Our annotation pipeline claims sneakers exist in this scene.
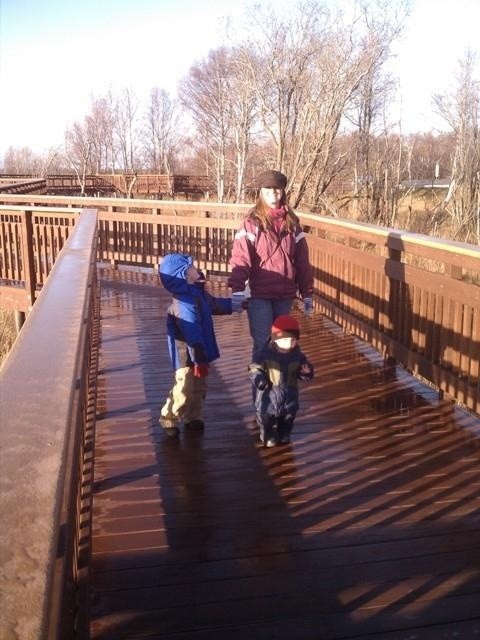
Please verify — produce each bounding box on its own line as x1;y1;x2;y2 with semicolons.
165;427;180;438
184;420;205;430
259;431;277;448
279;430;290;443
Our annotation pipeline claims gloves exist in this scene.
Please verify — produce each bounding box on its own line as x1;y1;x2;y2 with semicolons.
193;362;209;379
231;292;247;313
303;298;314;319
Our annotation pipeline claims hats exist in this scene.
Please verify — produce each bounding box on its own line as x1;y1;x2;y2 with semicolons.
256;170;287;190
270;315;300;341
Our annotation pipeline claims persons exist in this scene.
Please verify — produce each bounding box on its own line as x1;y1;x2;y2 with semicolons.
228;169;312;380
157;256;253;438
246;315;314;448
